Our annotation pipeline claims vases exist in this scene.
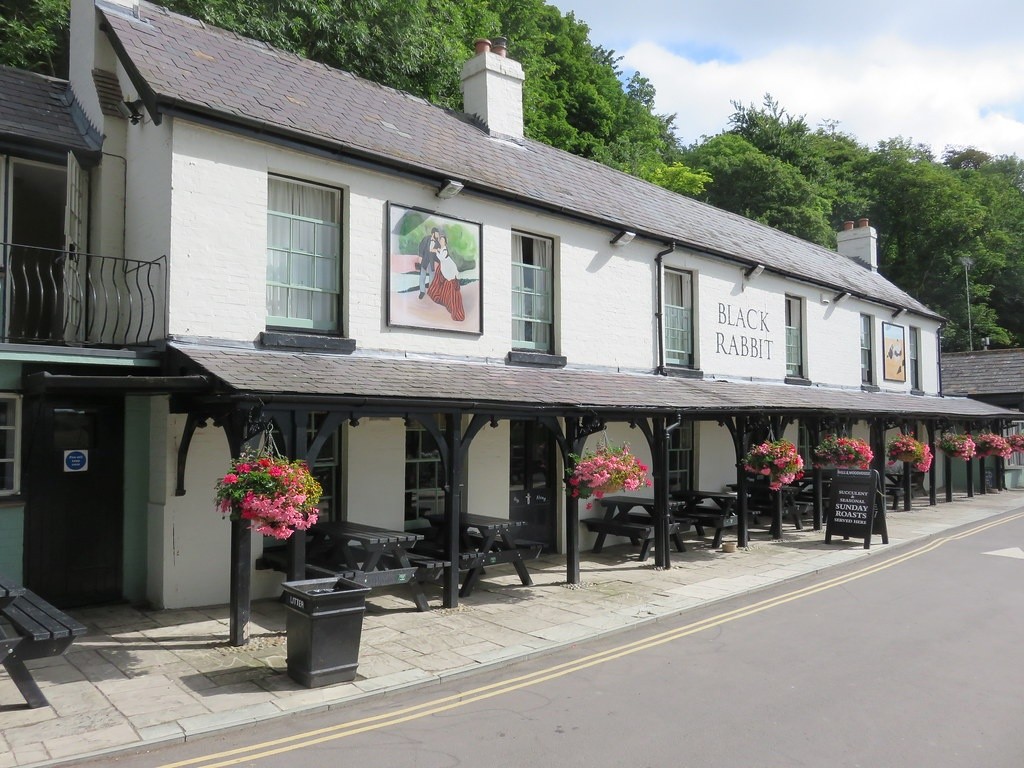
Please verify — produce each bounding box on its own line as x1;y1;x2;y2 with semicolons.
592;483;620;494
821;462;836;469
898;452;915;462
988;448;1002;455
946;450;961;457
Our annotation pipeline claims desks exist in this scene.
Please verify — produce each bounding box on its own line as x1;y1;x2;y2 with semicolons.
801;477;830;523
726;483;802;539
424;511;533;596
673;490;751;548
591;496;687;561
886;473;914;509
303;521;431;612
0;574;49;709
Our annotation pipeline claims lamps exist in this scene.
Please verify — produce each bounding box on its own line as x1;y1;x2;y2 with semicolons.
434;179;465;199
744;263;766;280
610;231;636;246
892;307;908;318
115;99;144;125
833;292;852;302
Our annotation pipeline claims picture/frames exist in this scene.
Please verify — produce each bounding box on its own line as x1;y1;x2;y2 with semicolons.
385;199;485;337
882;321;906;382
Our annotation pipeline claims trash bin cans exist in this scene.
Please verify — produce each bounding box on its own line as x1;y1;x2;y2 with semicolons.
281;576;372;688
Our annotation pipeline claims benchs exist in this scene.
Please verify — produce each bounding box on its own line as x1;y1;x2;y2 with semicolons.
885;486;904;492
798;496;830;504
673;510;724;528
255;546;363;581
0;588;87;662
911;483;917;488
793;501;813;512
696;505;763;515
408;539;483;570
748;503;789;516
465;530;545;561
629;512;698;524
581;517;655;539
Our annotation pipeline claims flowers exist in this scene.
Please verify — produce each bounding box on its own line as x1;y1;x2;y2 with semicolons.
934;429;976;463
812;432;874;470
563;438;652;509
212;442;324;542
1008;433;1024;454
976;432;1013;459
885;431;934;472
736;439;806;492
